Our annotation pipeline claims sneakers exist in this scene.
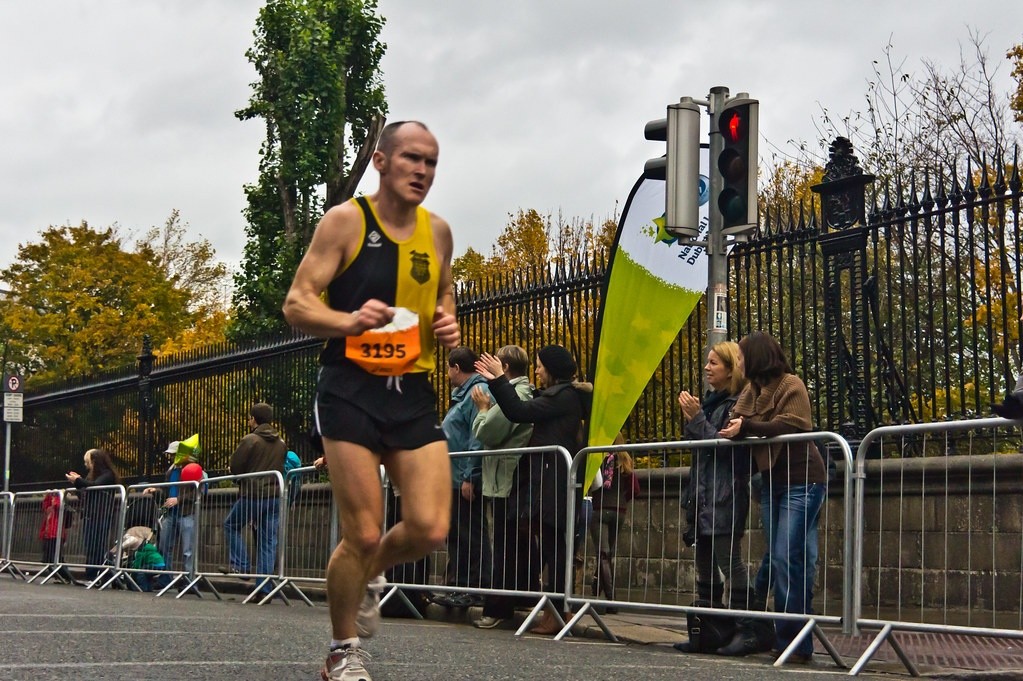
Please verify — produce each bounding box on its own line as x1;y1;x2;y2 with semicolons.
472;616;504;629
444;593;486;608
320;640;373;681
433;592;458;605
354;590;384;638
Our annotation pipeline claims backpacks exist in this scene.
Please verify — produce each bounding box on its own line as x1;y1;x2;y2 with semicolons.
283;450;302;504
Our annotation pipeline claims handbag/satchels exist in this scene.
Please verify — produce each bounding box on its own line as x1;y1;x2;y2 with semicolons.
684;599;738;654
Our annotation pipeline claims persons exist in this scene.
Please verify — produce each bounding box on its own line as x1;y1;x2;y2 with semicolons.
719;332;825;662
65;433;209;594
282;121;461;681
39;489;70;584
806;423;838;613
315;344;642;636
675;341;774;656
218;403;302;604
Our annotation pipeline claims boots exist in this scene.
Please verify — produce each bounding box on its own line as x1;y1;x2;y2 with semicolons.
596;551;618;614
672;581;725;652
590;567;604;596
715;586;760;657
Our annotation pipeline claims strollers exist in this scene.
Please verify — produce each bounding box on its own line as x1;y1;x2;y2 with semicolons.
90;491;174;592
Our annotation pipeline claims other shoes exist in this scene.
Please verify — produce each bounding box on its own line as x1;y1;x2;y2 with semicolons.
529;607;574;635
769;647;813;665
53;577;71;584
178;585;197;595
150;581;174;590
244;592;272;605
218;565;251;581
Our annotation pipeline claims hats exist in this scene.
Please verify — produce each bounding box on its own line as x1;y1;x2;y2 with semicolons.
537;345;577;379
164;440;180;454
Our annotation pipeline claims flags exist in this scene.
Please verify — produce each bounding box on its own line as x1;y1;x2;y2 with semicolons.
584;144;731;496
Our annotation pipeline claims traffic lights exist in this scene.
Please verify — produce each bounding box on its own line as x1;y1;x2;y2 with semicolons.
641;96;701;243
716;93;760;238
